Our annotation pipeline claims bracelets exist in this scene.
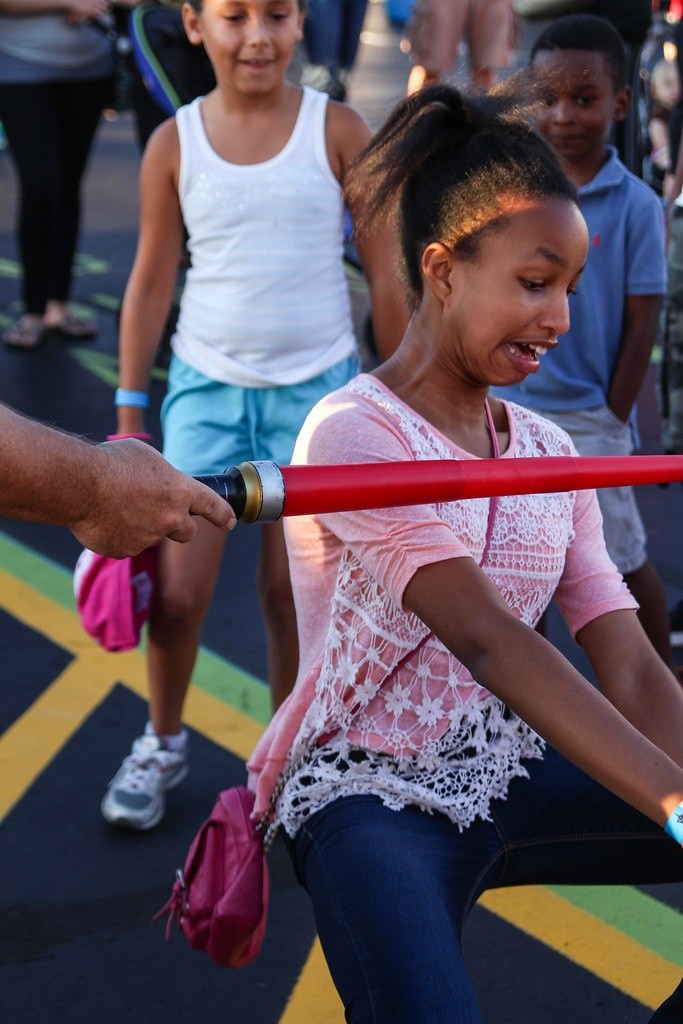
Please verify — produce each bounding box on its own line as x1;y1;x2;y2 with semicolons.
114;388;149;409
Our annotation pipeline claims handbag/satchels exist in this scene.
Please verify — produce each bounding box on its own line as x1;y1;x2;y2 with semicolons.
150;785;270;970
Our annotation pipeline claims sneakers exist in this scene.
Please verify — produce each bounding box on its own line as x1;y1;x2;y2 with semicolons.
668;599;683;647
100;722;199;831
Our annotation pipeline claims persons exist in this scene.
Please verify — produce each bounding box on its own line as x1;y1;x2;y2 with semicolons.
301;2;372;104
409;2;511;96
491;14;679;681
247;82;680;1022
1;404;237;559
93;2;418;827
1;0;119;351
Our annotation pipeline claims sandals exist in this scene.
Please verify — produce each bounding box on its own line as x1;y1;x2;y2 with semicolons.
3;315;44;350
45;317;93;341
